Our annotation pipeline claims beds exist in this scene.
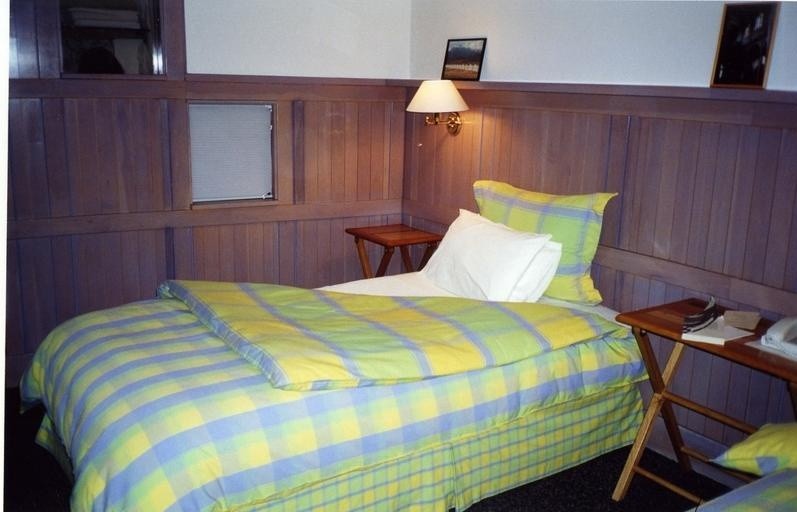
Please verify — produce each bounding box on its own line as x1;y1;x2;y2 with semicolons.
44;271;648;512
685;467;797;512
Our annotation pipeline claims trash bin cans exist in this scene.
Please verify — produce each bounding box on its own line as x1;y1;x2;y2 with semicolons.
761;315;797;360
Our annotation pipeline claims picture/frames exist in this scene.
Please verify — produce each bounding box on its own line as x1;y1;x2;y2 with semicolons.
708;0;781;89
440;37;487;82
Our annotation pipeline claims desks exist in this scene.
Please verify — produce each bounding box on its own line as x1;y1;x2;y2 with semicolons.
611;296;797;503
344;222;444;279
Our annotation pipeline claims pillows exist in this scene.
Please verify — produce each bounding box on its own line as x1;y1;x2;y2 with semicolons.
708;419;797;476
421;179;619;305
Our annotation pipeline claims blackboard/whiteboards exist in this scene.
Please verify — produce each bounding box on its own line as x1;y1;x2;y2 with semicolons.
186;102;275;204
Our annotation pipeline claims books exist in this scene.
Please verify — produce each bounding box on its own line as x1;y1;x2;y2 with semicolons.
680;294;726;348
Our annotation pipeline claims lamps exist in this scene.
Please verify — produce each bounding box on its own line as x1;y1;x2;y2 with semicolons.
406;80;470;136
